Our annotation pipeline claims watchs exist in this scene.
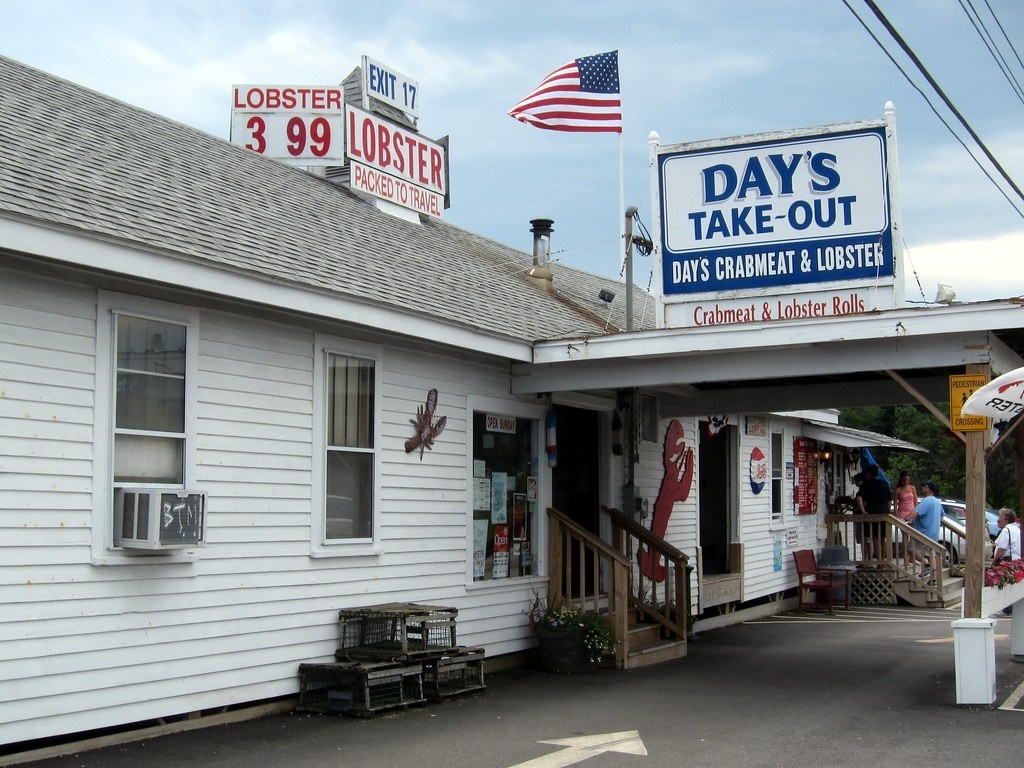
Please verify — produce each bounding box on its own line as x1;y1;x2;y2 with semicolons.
904;517;908;522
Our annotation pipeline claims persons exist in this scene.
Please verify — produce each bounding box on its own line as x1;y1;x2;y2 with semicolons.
893;470;918;567
852;465;892;570
991;508;1021;566
901;482;946;589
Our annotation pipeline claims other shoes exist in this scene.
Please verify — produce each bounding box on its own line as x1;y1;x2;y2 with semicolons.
927;580;937;586
919;566;936;577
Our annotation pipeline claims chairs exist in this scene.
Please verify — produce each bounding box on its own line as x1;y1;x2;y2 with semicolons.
793;550;852;614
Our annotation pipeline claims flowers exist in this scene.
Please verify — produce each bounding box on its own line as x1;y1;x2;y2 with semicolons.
984;557;1024;589
521;578;622;663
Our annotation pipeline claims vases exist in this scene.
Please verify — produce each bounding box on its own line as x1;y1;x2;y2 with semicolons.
535;630;599;674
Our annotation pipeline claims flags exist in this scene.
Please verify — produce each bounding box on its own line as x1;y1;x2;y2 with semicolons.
507;49;621;133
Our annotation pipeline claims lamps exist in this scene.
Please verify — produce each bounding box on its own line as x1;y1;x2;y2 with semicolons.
818;443;833;464
850;447;862;470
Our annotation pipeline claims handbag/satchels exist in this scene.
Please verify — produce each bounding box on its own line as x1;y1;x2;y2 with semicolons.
996;556;1012;566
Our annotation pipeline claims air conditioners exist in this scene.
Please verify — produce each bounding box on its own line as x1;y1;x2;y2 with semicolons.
115;487;207;548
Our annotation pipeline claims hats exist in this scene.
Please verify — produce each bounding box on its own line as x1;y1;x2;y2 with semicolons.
920;480;936;492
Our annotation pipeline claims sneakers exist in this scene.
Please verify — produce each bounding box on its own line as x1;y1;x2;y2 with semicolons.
995;611;1013;618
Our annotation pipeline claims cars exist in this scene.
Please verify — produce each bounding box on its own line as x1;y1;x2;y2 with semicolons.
888;498;1021;564
